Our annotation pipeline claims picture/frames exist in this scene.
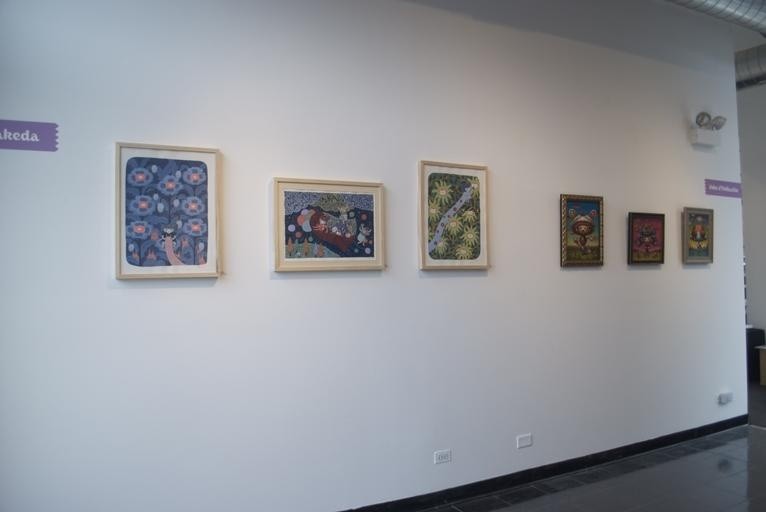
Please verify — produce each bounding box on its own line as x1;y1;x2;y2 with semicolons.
682;207;714;267
114;139;222;282
628;211;666;265
561;191;606;268
272;174;387;273
418;159;494;271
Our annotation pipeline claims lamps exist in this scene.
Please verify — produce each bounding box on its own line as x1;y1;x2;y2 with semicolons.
687;109;729;149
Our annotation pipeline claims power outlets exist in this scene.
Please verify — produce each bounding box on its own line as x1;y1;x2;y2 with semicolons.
431;446;453;466
